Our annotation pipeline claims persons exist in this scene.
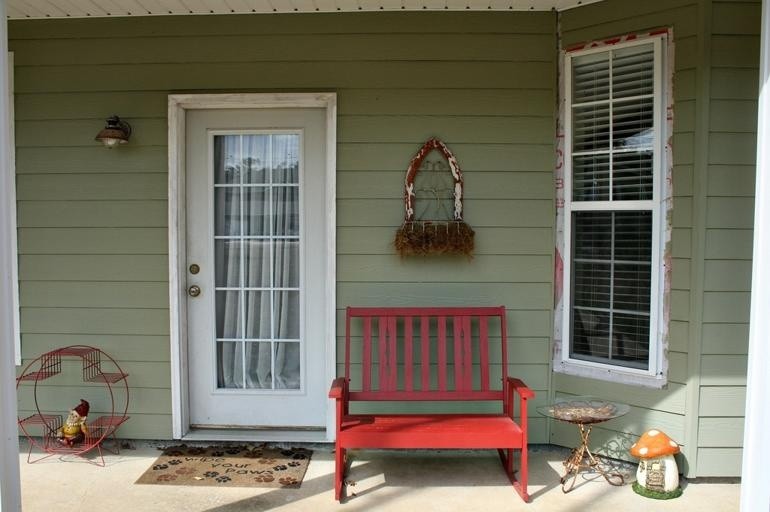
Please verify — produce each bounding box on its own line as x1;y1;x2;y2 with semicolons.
55;399;88;447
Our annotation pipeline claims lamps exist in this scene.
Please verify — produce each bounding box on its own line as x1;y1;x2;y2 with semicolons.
94;116;131;148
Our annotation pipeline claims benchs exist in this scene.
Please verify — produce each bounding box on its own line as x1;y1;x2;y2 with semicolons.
326;305;535;503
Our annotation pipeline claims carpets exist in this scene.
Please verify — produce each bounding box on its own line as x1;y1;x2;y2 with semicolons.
133;444;316;489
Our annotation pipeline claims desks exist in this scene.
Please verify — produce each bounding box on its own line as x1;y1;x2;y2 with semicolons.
537;398;631;494
